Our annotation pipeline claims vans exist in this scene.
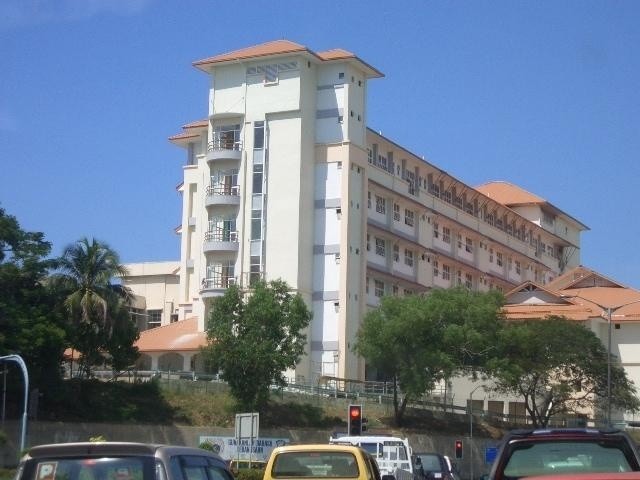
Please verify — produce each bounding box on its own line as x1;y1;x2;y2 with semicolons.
443;456;461;480
15;439;235;480
263;444;396;480
229;459;266;471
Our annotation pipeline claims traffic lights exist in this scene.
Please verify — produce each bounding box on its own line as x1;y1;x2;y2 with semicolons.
455;440;463;460
361;417;368;434
348;405;362;436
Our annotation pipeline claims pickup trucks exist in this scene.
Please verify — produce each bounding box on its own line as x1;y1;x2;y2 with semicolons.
480;426;640;480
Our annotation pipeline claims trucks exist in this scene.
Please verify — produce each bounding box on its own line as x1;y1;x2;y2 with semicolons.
299;433;414;480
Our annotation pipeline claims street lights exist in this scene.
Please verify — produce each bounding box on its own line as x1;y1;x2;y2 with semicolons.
558;293;640;429
469;383;489;480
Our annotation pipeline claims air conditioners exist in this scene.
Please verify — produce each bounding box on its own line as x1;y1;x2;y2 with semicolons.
231;186;239;196
230;232;238;242
228;278;236;288
233;141;242;151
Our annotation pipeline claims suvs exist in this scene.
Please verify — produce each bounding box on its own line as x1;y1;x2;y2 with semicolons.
413;451;456;480
